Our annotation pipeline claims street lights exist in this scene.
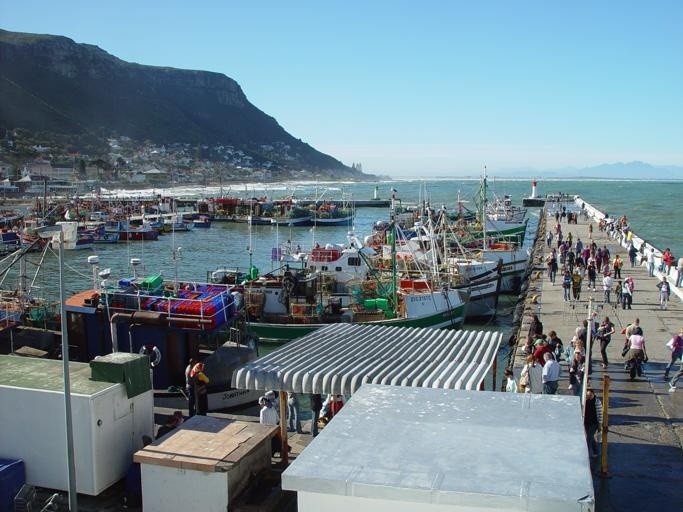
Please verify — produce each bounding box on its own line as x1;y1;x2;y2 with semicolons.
35;225;79;509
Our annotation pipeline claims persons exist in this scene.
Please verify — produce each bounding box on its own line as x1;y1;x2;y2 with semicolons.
157;411;184;439
501;191;682;459
184;357;197;419
190;362;210;417
257;390;346;459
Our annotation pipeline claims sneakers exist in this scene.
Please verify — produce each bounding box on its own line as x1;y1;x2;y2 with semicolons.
669;382;676;389
601;363;608;369
568;384;573;389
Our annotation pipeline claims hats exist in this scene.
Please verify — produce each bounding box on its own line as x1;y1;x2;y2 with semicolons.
534;339;542;346
571;337;579;342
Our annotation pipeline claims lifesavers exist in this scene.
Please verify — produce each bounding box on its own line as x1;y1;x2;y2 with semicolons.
138;344;161;368
